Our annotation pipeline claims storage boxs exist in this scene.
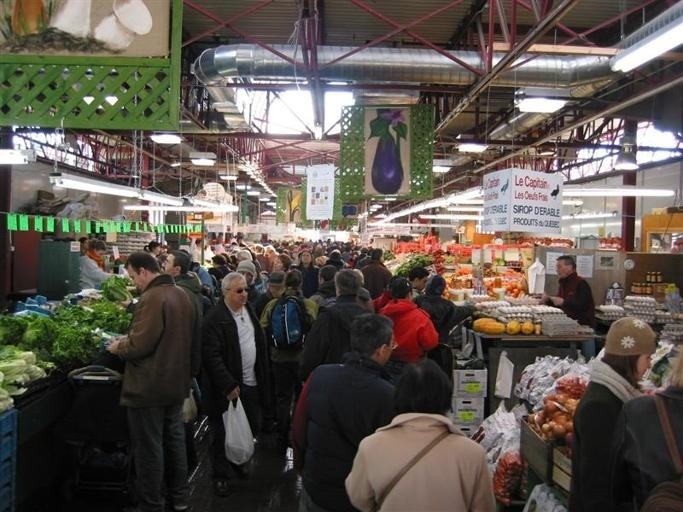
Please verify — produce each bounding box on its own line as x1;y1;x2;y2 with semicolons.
449;346;489;442
518;418;552;484
551;444;573;497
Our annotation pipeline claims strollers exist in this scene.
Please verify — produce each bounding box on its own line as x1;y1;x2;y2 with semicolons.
56;363;137;502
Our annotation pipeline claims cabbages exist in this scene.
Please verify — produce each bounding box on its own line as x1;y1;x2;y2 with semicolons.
100;275;133;304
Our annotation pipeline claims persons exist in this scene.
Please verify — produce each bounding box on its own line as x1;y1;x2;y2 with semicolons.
567;317;656;512
607;344;683;512
538;256;596;364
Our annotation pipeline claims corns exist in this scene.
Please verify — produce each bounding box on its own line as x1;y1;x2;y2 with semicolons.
506;321;521;335
472;318;495;331
479;322;504;334
522;322;533;335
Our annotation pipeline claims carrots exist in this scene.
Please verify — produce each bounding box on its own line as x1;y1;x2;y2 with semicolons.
557;377;585;399
492;453;524;506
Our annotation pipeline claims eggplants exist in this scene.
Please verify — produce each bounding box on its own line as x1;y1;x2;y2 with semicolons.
371;131;405;195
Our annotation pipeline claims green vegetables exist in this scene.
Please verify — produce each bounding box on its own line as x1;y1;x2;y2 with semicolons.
0;343;49;414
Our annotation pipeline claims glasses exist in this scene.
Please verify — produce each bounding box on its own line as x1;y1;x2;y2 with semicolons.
236;288;251;294
385;342;398;350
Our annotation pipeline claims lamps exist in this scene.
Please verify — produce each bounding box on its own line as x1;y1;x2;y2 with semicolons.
514;29;570;113
563;200;583;206
615;144;641;170
0;130;277;212
563;188;674;197
432;145;450;173
609;1;682;73
457;86;490;153
431;189;484;211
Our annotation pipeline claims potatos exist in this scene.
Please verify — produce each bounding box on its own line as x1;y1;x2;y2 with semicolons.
526;393;578;459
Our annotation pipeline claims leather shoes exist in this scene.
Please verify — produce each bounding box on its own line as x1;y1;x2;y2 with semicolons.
212;466;233;497
236;465;249;480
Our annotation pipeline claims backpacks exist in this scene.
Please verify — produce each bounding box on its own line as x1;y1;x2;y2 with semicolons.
267;295;312;353
639;393;683;512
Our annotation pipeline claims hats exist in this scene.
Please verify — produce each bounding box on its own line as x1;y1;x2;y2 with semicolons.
236;259;255;277
604;316;656;356
